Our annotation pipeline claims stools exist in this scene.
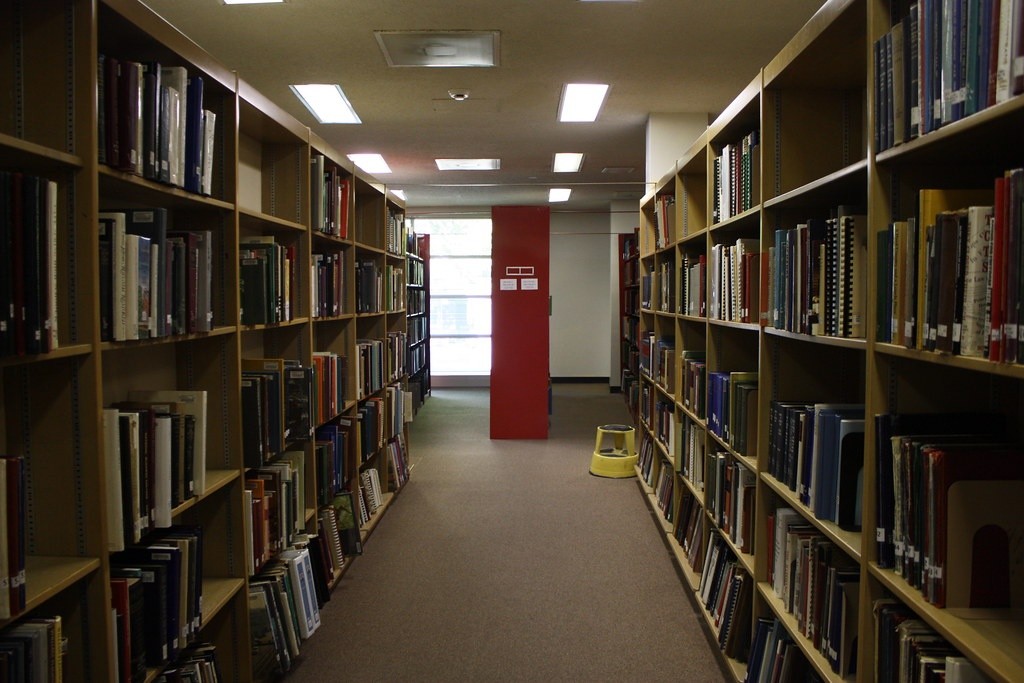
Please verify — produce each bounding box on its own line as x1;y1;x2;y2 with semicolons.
590;424;639;478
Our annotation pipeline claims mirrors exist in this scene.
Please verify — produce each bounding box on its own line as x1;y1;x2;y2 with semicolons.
0;0;1024;683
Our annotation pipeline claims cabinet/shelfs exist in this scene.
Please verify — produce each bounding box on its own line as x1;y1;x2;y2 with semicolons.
0;0;431;683
617;0;1024;683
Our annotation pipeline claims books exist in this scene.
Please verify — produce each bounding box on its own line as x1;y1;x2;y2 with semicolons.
0;54;430;683
621;0;1023;683
